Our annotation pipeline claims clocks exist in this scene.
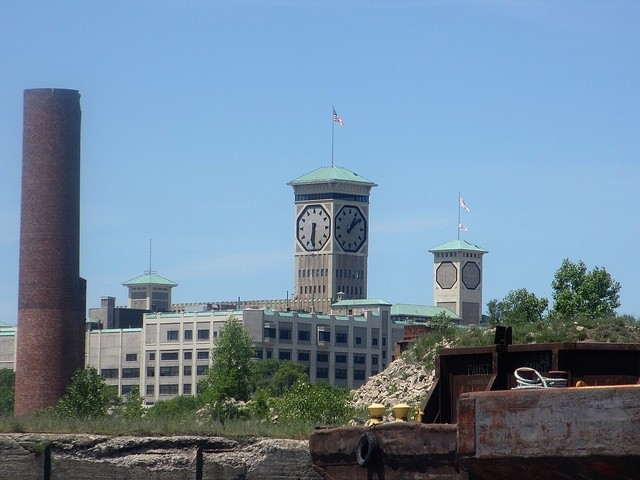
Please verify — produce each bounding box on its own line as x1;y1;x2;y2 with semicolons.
335;206;367;252
296;202;331;252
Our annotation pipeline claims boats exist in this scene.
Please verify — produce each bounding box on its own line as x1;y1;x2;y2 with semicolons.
309;326;640;480
